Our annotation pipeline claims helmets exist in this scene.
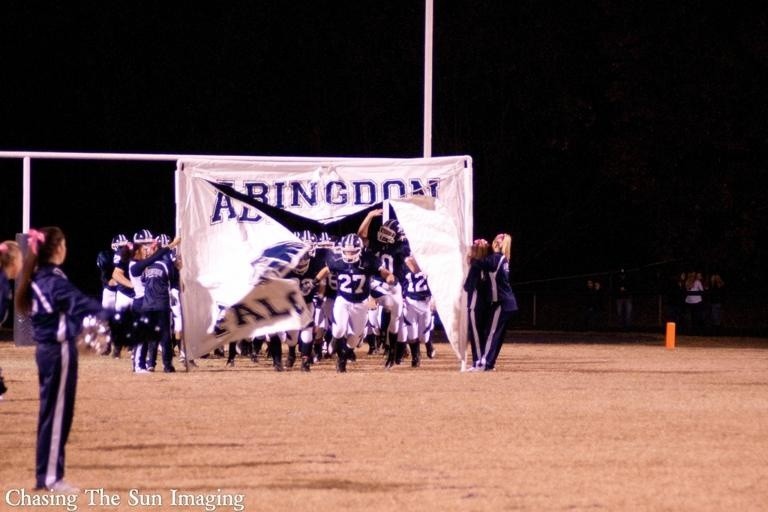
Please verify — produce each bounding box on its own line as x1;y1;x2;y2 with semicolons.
377;219;402;243
111;229;170;250
292;230;363;274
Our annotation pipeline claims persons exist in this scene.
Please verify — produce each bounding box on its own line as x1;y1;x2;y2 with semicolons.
16;227;111;493
0;239;23;398
582;268;728;329
92;209;522;375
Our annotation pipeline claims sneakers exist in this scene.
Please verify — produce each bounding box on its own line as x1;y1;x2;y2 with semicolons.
47;481;80;497
